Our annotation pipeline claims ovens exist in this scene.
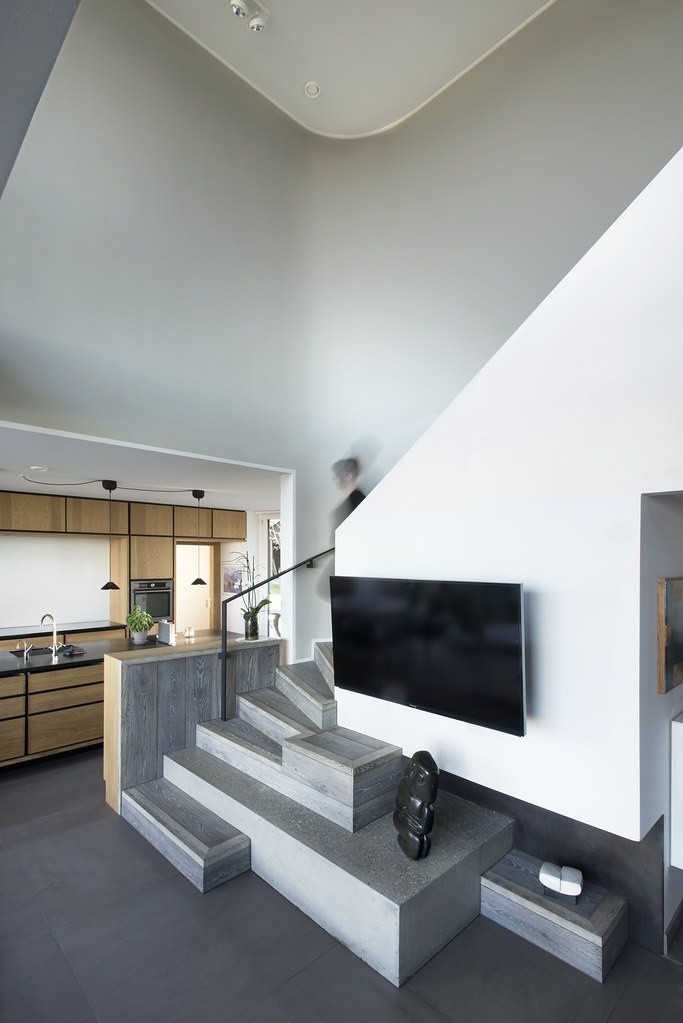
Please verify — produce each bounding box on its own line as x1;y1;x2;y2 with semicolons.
130;579;174;623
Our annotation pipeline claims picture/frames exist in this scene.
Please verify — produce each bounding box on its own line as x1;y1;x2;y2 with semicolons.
220;563;245;596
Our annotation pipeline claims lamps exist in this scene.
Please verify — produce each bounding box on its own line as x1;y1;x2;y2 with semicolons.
230;0;270;31
191;490;207;586
100;480;120;590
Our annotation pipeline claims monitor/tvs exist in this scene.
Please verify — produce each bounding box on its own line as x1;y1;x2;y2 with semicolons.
328;576;526;738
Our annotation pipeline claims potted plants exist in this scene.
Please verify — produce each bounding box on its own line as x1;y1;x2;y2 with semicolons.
217;551;272;640
126;606;155;644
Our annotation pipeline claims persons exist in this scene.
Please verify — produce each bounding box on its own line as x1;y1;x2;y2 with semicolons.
314;458;366;603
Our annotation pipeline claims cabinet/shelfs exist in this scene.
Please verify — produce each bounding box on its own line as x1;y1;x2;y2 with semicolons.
0;491;248;780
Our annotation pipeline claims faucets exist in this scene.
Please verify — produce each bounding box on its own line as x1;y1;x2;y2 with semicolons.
40;614;58;656
16;639;30;659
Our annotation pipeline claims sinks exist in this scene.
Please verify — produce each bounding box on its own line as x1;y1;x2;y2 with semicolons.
9;644;75;657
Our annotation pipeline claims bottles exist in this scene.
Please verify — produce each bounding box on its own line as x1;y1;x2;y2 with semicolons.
183;627;194;637
